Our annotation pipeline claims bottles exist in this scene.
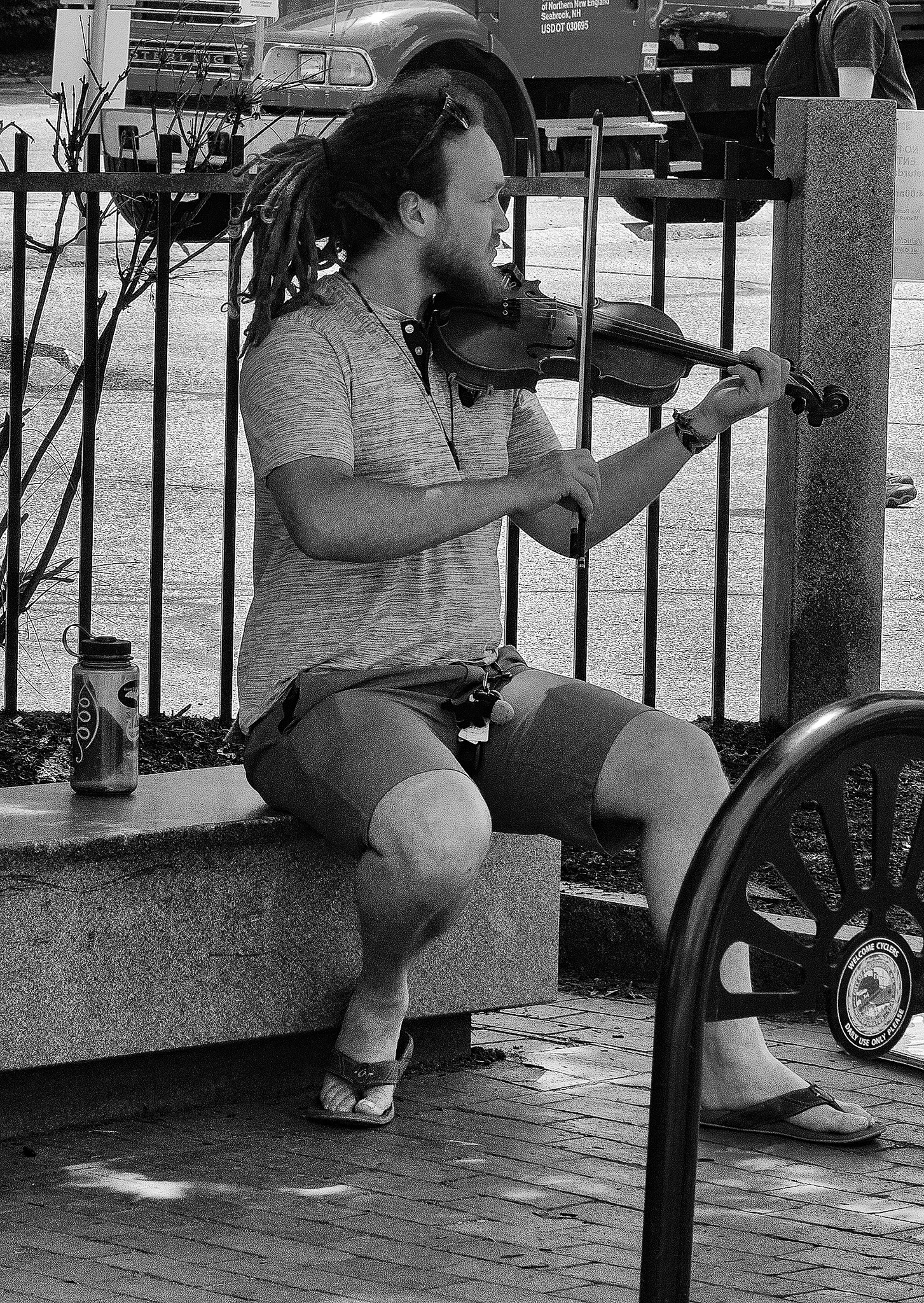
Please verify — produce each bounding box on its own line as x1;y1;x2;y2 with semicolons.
62;621;141;796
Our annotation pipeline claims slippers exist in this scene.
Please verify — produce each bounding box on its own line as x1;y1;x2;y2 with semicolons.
698;1077;887;1144
305;1029;414;1125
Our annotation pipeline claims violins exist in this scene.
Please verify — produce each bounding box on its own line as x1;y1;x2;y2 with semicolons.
430;261;850;425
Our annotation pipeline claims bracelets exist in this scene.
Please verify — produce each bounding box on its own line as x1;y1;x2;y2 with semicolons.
672;406;716;455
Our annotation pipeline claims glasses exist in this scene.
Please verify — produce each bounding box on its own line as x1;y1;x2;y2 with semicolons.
394;92;470;185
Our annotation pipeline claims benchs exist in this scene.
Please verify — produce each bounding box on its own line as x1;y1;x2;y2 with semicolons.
0;763;560;1146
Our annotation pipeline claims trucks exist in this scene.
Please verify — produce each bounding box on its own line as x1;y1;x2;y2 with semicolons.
103;0;924;244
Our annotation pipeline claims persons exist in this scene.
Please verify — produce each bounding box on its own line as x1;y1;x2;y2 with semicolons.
229;91;887;1146
